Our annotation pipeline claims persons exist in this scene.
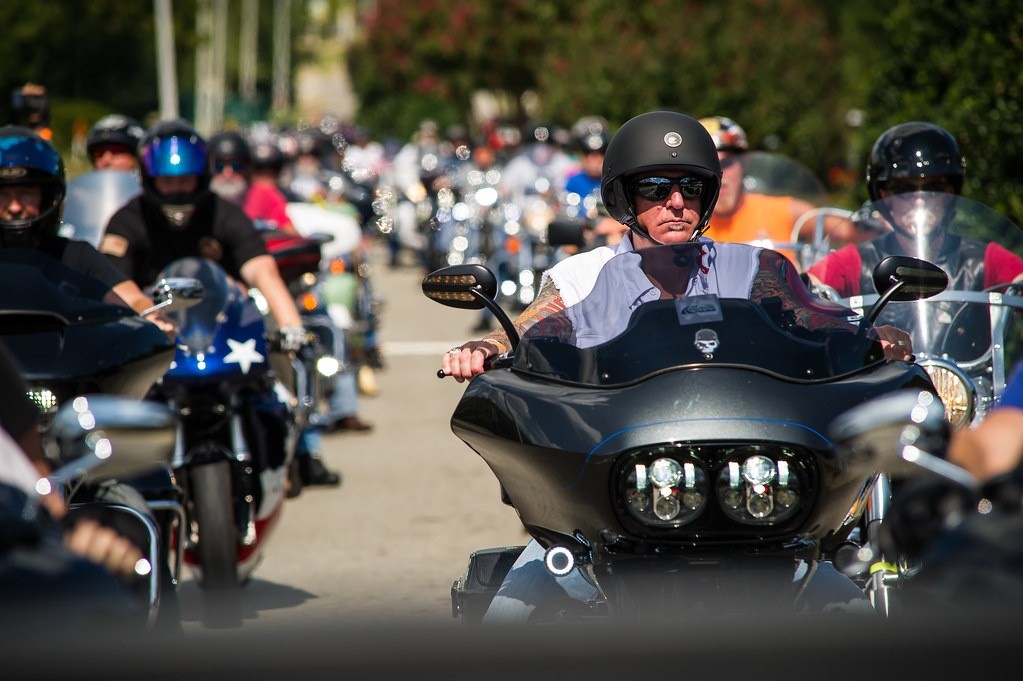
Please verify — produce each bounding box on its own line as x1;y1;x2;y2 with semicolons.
0;90;1023;637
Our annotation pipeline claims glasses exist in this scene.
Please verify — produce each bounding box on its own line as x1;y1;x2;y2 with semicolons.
883;180;952;199
214;163;248;175
635;175;708;201
721;156;735;169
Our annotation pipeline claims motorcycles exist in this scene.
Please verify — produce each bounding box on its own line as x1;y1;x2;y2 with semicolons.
793;190;1022;432
0;134;864;680
421;239;952;678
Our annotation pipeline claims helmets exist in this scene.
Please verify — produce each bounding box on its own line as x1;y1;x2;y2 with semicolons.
87;114;145;167
865;121;964;208
257;123;356;168
0;125;65;249
207;132;252;164
138;124;212;228
697;115;750;156
488;118;611;162
601;111;721;221
